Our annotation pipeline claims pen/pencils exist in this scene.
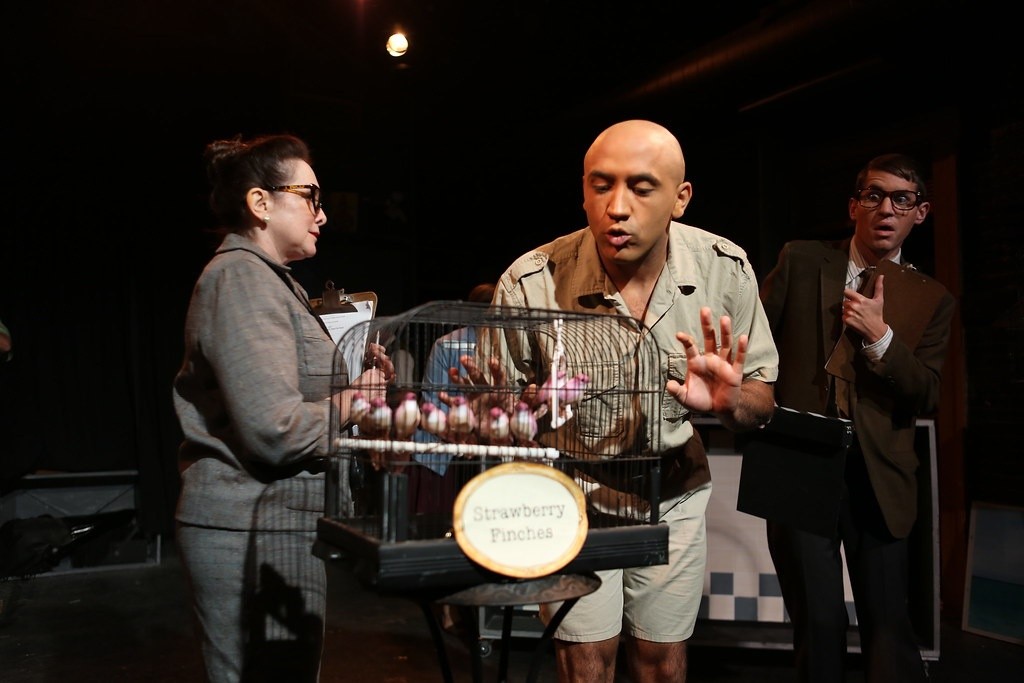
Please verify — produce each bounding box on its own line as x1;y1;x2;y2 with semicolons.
372;330;379;369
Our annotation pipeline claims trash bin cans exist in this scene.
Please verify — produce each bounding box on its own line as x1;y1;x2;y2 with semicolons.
0;487;165;683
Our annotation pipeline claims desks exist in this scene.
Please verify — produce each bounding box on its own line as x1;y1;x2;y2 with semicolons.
1;486;135;575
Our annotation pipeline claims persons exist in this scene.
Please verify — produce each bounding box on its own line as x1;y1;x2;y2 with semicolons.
173;133;397;683
413;120;779;683
737;153;956;683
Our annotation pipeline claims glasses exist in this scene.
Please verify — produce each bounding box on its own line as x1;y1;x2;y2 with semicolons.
261;184;322;208
856;188;922;210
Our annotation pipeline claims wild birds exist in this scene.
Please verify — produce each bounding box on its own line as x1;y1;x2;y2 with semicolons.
349;371;588;445
